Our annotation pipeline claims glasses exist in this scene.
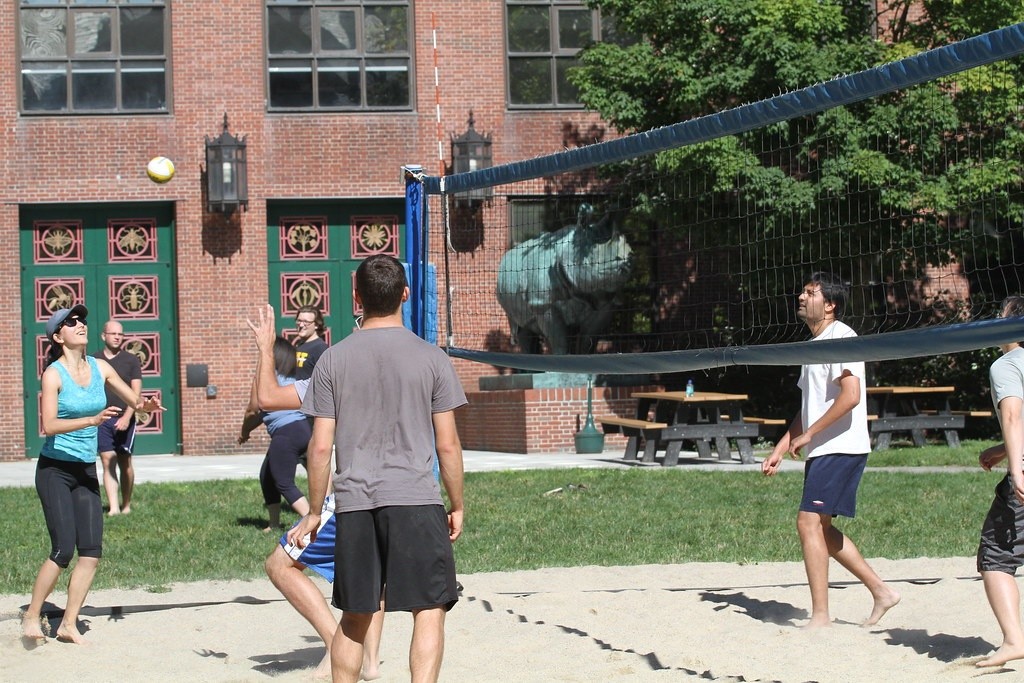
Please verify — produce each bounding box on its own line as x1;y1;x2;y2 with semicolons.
104;333;124;337
295;318;315;326
56;317;87;334
997;312;1004;320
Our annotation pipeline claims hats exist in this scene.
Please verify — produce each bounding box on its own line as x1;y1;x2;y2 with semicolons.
45;304;88;340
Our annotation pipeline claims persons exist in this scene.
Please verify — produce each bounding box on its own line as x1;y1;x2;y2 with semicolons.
246;304;385;680
975;295;1024;668
763;272;899;629
238;337;310;533
93;320;141;516
294;306;328;380
21;304;167;645
285;254;469;683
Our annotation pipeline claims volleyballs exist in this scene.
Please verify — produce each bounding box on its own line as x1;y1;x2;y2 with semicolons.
146;156;175;184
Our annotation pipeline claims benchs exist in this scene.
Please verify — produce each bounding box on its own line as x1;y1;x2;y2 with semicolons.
867;409;992;452
595;415;788;468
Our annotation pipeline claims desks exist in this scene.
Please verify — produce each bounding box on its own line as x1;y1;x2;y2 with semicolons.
866;385;965;452
624;391;760;468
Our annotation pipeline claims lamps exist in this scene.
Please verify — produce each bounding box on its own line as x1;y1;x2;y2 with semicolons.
450;110;495;209
204;111;249;224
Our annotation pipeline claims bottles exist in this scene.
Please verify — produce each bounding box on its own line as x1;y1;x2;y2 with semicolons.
686;380;693;397
